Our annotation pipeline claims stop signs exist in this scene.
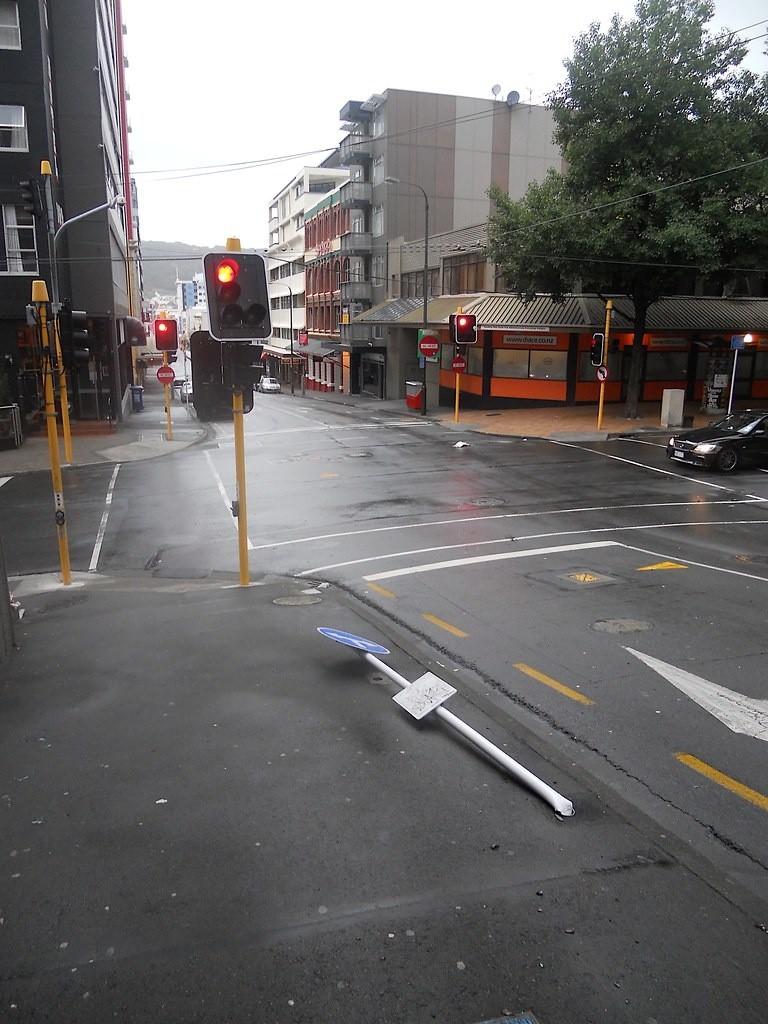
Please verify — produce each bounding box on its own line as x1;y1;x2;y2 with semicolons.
451;358;466;373
417;329;441;358
158;367;174;383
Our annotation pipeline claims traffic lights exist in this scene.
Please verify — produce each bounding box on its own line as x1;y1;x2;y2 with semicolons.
591;333;603;366
20;178;44;218
154;319;178;351
455;314;478;344
203;252;273;341
64;298;90;374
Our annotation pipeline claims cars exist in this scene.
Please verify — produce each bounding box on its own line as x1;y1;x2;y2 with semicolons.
173;371;188;386
666;411;768;474
179;382;193;405
252;383;257;391
259;378;282;394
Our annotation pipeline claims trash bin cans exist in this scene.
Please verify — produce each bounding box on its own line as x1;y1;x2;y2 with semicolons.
406;381;423;412
0;402;24;451
130;385;144;410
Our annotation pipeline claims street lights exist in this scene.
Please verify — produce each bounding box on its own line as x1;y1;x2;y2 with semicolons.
385;176;429;415
